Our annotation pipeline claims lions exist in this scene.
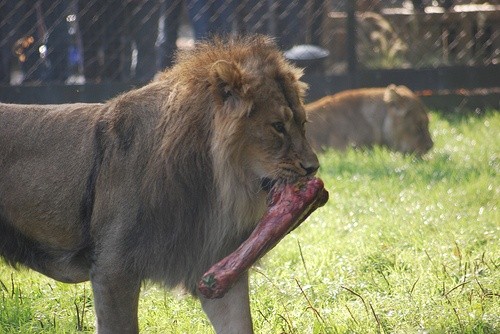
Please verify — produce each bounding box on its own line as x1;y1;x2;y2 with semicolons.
305;83;433;154
0;35;329;334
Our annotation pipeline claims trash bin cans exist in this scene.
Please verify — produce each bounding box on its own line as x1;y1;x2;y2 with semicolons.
284;44;329;99
